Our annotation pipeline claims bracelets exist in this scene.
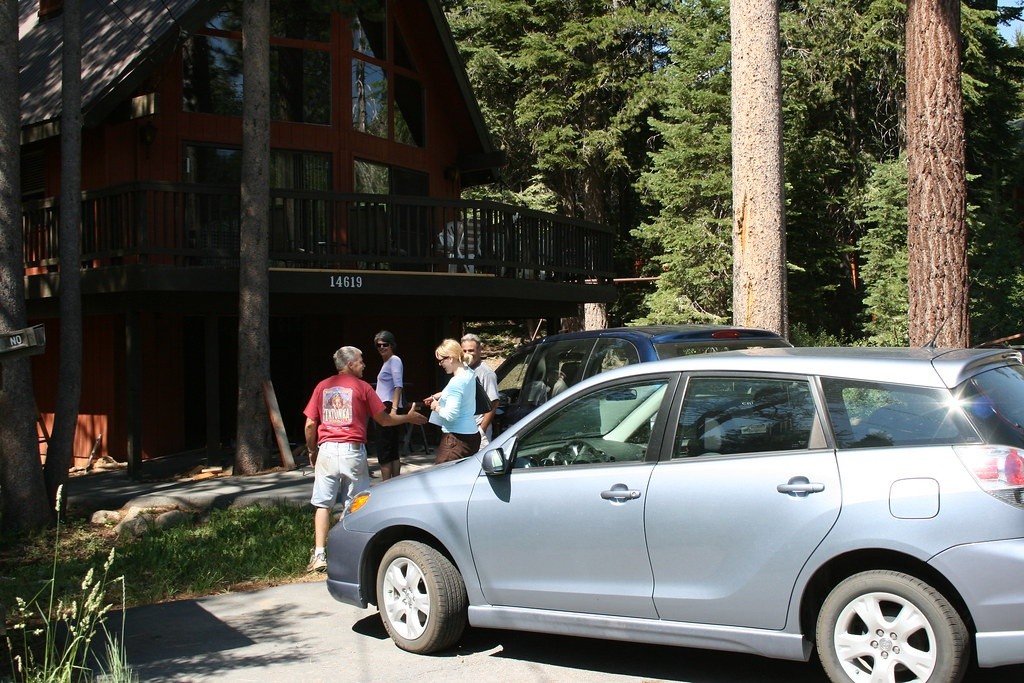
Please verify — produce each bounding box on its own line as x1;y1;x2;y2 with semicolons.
391;406;397;411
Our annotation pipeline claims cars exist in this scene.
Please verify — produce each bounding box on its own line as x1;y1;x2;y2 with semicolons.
323;343;1024;683
488;323;799;445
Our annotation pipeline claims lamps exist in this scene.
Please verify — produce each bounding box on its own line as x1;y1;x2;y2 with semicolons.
139;119;158;145
444;160;460;190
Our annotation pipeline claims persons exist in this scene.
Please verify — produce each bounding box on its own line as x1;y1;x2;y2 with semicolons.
303;346;428;572
423;333;501;451
552;363;577;399
375;331;404;480
428;339;482;464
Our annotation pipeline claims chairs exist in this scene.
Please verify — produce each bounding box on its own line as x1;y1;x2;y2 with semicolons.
430;217;481;274
751;387;787;423
551;362;581;398
691;409;739;457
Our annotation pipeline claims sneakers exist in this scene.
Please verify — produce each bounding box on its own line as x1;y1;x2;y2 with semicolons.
306;548;327;573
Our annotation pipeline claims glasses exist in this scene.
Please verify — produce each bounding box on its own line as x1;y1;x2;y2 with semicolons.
438;357;448;362
376;343;389;347
